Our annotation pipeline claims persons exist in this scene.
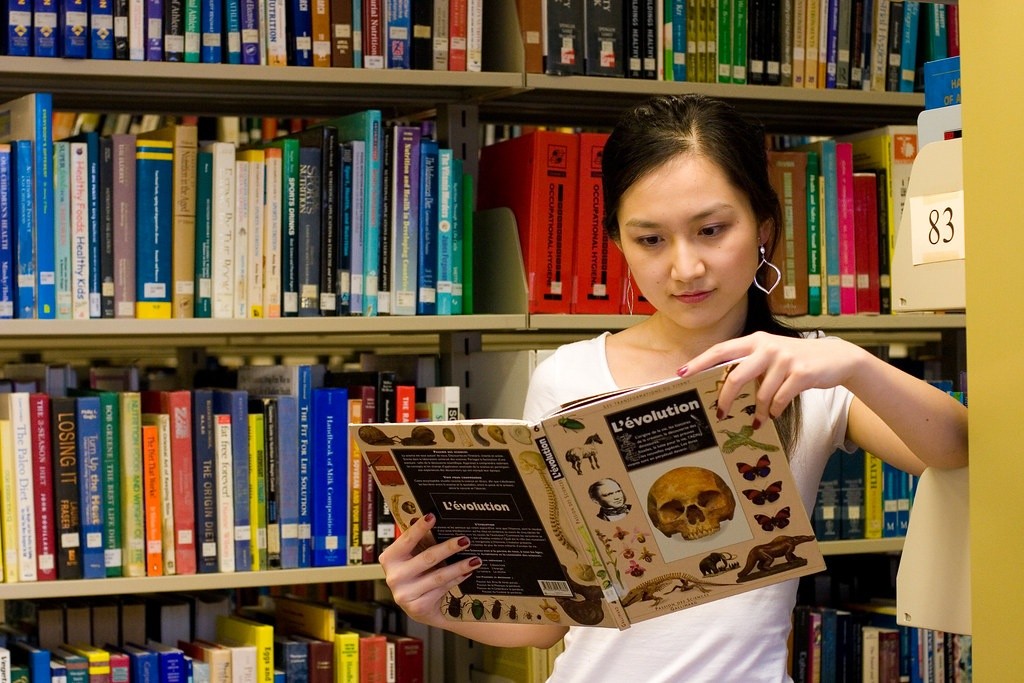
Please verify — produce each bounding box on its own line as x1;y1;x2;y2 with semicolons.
587;478;632;521
380;93;970;683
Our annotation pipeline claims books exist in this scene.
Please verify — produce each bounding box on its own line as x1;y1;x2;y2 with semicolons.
0;0;971;683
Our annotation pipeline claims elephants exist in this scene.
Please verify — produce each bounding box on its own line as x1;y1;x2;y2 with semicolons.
699;551;738;576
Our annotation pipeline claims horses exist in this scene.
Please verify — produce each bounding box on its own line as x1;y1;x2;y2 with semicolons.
564;432;604;476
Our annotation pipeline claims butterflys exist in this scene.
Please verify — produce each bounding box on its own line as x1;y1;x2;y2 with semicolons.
735;452;792;532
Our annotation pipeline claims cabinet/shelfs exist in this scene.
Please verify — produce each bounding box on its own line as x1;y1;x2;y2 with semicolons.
0;0;975;683
